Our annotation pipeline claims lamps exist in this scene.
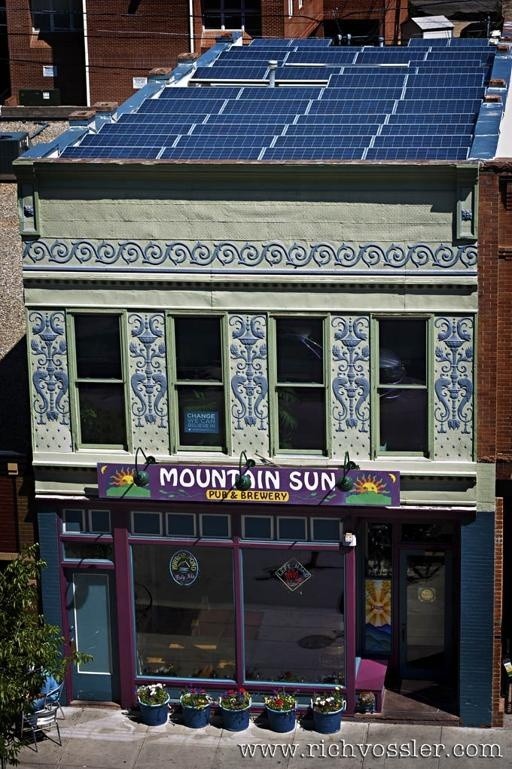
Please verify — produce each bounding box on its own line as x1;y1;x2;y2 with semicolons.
236;451;255;489
134;447;156;485
338;451;360;490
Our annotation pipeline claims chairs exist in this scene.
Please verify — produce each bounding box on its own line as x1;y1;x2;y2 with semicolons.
21;682;65;752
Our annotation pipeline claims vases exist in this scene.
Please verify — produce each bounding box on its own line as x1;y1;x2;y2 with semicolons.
130;695;346;734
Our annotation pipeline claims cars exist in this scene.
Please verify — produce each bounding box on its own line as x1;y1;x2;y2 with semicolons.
276;324;407;403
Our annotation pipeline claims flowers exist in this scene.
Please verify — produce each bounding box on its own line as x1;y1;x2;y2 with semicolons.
136;683;344;715
